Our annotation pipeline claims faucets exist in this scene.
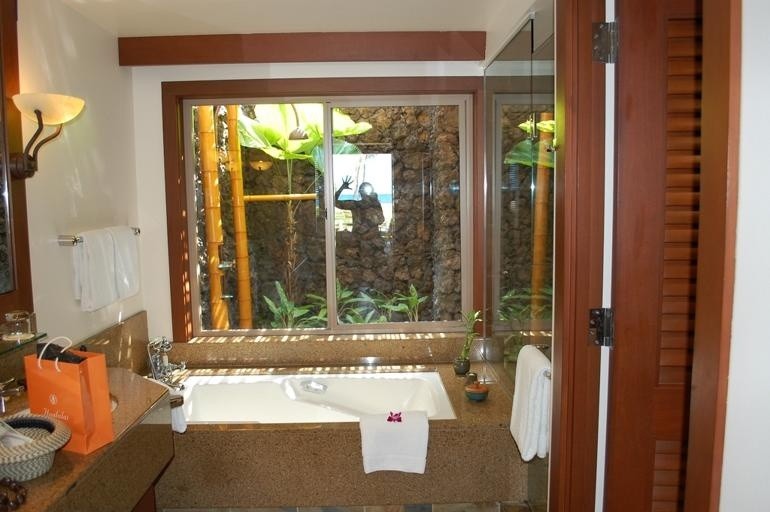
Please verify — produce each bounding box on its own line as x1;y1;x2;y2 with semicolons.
0;376;25;412
166;380;185;390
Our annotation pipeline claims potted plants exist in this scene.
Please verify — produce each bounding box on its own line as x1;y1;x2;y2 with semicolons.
452;309;483;373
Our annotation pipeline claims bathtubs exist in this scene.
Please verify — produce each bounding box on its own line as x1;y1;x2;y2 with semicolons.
171;372;457;425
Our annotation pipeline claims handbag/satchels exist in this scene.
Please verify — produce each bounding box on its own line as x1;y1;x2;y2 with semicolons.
20;335;116;457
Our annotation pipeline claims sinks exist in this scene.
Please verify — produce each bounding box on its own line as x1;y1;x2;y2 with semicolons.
12;392;119;414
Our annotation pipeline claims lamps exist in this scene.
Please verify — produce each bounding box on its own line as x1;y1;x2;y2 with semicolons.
9;92;86;181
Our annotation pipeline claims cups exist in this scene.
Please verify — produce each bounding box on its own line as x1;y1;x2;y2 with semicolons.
5;310;30;339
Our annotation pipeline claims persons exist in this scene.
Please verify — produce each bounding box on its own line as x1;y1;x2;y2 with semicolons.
335;175;385;238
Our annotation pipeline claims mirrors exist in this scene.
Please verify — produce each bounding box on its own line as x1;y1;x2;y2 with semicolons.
1;0;34;321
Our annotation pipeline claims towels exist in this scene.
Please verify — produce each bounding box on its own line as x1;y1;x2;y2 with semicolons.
359;411;429;475
146;375;188;434
510;343;554;464
72;229;117;312
103;226;143;300
